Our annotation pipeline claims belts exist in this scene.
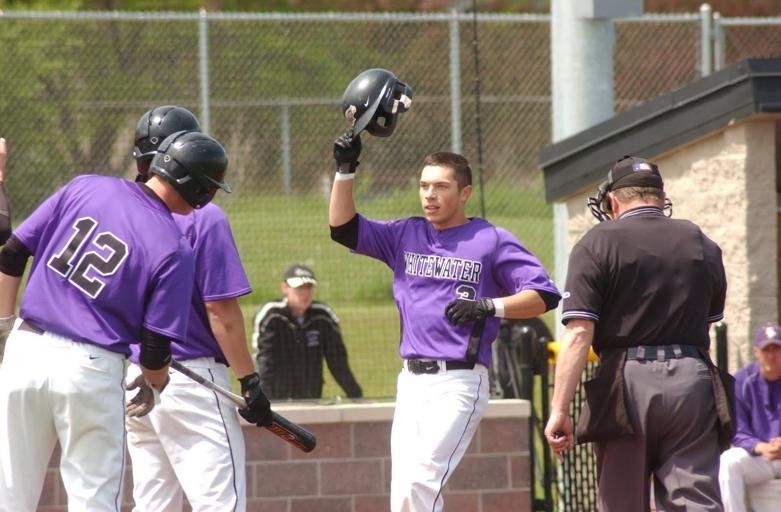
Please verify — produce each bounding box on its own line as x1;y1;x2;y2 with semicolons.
407;357;475;374
624;345;689;360
18;321;91;342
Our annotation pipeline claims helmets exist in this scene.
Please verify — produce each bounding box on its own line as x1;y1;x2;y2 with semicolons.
147;130;233;209
586;154;673;223
132;104;201;182
342;68;413;138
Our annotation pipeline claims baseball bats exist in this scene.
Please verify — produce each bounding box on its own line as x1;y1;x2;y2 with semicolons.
168;358;318;453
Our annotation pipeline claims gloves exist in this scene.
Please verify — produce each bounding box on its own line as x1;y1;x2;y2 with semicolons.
126;375;171;419
444;297;495;326
332;128;362;174
237;371;273;428
1;315;17;361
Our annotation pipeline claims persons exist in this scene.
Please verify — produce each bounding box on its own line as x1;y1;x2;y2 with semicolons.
0;137;13;246
249;263;363;404
715;320;781;511
328;129;563;512
544;153;728;511
122;105;270;512
0;130;233;512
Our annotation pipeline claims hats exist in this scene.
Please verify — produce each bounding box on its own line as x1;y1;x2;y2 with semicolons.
284;264;318;289
753;322;780;351
